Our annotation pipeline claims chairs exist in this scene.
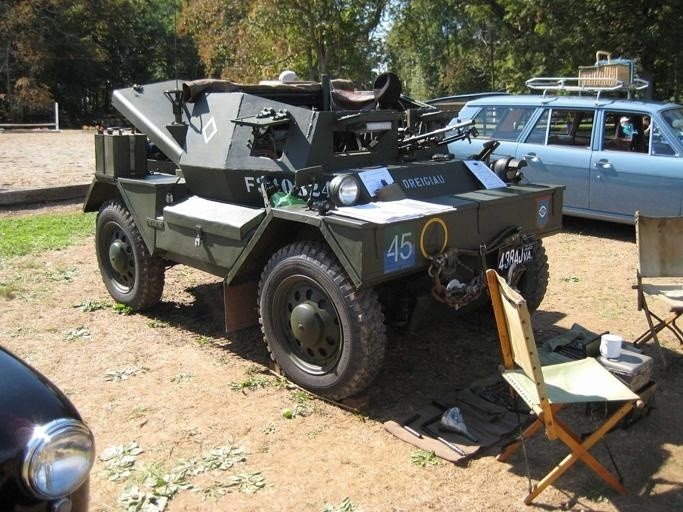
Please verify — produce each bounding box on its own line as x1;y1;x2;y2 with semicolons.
632;208;683;374
482;267;646;505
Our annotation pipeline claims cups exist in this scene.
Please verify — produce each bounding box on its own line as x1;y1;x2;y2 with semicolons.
599;334;622;359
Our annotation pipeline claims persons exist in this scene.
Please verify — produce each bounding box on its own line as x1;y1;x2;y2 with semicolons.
640;115;654;139
566;117;576;138
619;115;636;138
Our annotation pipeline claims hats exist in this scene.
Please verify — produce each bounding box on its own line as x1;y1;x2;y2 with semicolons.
619;116;629;122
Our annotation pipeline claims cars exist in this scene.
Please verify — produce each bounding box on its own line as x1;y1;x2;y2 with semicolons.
0;341;99;512
439;74;682;227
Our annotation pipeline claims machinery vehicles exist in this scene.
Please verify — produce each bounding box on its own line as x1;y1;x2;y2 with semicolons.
77;67;572;402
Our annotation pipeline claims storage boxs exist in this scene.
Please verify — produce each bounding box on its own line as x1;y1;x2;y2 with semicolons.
585;348;659;427
